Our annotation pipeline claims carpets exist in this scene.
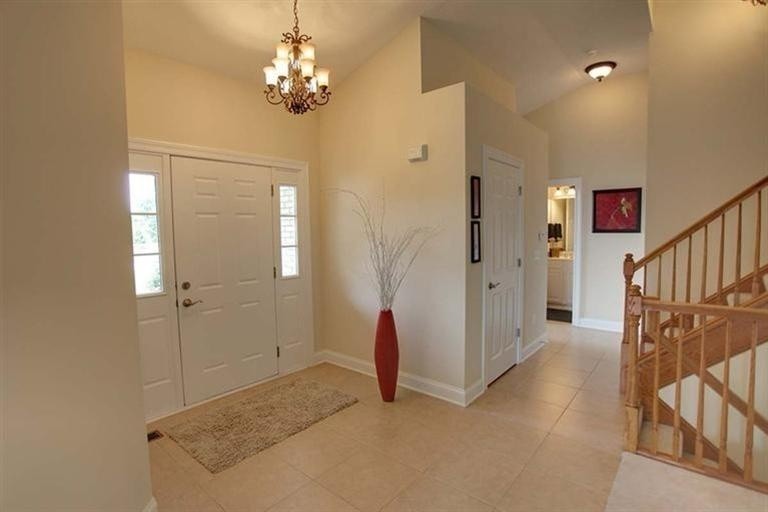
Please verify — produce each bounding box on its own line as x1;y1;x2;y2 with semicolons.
165;377;359;475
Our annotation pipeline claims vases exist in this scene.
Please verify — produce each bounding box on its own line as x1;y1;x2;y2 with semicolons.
373;309;401;402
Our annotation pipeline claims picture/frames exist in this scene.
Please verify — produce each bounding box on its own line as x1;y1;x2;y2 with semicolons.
471;221;481;263
591;186;643;234
470;174;482;219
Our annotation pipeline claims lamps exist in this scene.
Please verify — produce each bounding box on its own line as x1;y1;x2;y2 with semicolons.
567;186;576;195
555;187;563;196
262;0;332;115
584;60;617;82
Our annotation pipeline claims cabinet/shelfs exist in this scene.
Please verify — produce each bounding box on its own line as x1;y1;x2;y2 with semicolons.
547;260;573;311
